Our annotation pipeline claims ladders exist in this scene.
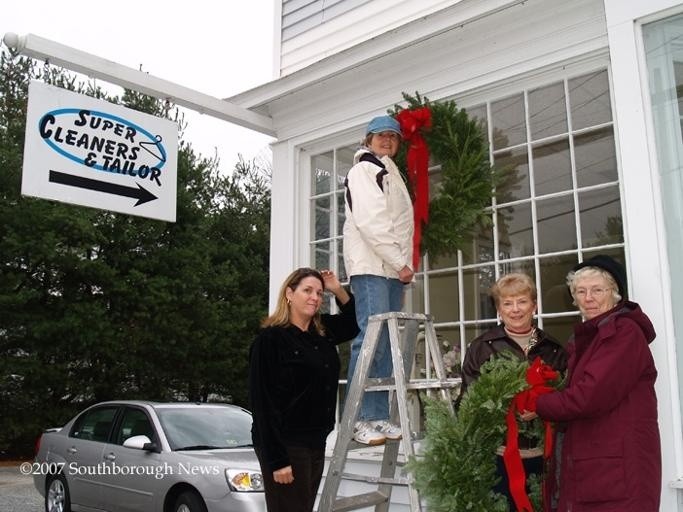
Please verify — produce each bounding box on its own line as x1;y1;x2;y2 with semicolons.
317;311;462;512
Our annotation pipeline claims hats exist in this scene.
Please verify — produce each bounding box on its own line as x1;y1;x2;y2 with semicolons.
365;116;403;137
573;254;627;303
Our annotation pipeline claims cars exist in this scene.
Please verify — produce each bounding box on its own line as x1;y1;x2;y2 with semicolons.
31;400;266;511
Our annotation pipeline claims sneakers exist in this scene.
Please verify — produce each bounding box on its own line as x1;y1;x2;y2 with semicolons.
369;418;405;441
352;420;388;446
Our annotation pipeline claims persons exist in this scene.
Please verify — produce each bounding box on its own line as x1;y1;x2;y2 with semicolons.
343;116;406;446
249;268;357;511
520;251;661;512
450;271;567;511
